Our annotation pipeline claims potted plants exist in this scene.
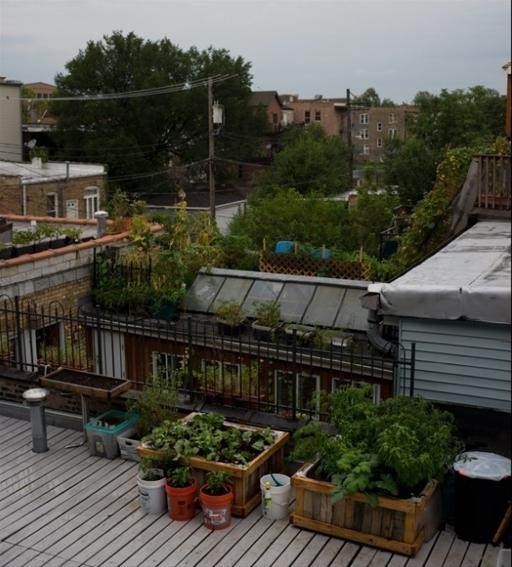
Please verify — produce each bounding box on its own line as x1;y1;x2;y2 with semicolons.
116;249;193;323
0;220;86;261
208;292;359;353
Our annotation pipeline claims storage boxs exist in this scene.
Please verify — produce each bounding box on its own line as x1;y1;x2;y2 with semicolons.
83;409;139;461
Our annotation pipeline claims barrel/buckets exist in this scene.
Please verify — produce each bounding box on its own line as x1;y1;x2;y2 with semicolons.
260;473;295;520
199;483;234;530
165;478;199;521
136;473;165;514
